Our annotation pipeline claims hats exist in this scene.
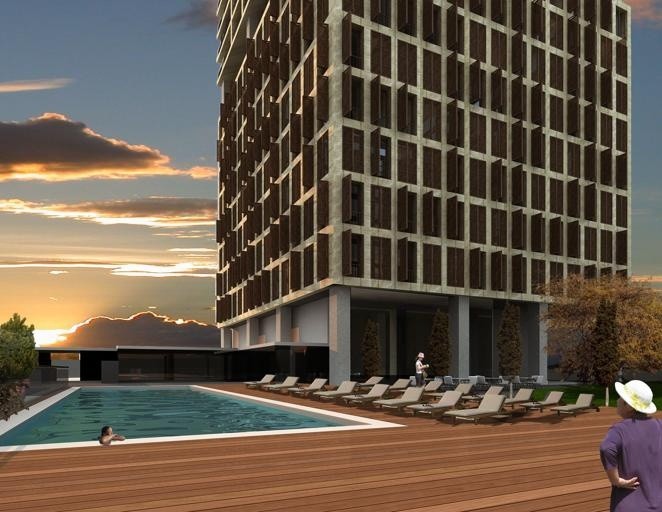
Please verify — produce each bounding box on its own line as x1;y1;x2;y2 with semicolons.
615;380;657;415
418;352;425;358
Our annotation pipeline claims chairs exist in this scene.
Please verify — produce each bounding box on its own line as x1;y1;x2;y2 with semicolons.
406;390;463;418
369;379;412;395
356;376;384;392
431;383;474;400
312;380;359;401
341;384;390;409
264;376;301;393
288;377;328;397
244;374;275;389
444;394;512;426
550;393;599;418
462;385;505;406
400;381;443;395
373;387;429;413
504;388;536;409
519;391;565;413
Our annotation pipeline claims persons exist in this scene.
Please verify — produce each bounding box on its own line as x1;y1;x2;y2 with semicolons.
415;352;429;387
600;380;662;512
100;426;125;444
